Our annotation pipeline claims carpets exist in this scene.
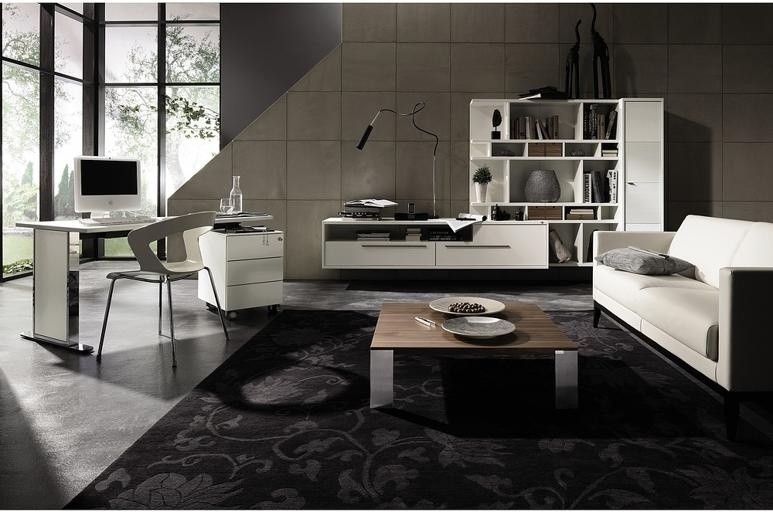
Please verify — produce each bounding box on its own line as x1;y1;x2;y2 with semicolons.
62;309;773;510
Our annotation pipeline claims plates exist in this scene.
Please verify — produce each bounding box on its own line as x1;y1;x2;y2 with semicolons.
441;316;516;339
429;296;506;315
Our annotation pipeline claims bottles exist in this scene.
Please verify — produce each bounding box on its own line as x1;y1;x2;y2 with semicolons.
492;207;497;219
229;176;243;214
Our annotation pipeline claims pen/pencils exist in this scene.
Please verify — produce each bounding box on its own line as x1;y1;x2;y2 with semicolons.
456;218;476;221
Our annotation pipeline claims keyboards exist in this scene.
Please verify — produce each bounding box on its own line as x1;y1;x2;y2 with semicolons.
79;216;157;226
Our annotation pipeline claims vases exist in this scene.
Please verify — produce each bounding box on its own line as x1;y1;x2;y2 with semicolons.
475;181;488;202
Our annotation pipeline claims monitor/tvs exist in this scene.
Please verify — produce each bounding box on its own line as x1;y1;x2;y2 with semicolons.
73;156;141;217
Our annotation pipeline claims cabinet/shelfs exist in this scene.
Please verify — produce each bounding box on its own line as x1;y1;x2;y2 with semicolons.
322;97;665;270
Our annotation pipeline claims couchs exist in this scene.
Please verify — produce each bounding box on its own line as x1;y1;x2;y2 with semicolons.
592;215;773;383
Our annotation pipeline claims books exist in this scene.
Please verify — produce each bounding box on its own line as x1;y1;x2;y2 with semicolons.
428;213;487;234
567;208;594;220
345;198;399;208
607;169;618;204
426;230;457;241
583;173;592;203
508;113;560;140
592;171;604;203
583;111;618;139
404;227;422;241
356;227;390;241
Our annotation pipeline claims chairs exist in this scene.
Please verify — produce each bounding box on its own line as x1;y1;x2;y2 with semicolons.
97;212;229;368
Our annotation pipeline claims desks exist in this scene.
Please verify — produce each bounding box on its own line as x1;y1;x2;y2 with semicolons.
15;211;284;353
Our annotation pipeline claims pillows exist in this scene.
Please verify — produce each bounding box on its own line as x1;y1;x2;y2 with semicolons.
595;246;693;276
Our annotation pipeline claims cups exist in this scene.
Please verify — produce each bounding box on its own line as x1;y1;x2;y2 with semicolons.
219;198;233;213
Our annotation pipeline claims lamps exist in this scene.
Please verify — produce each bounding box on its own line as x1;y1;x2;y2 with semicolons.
356;100;439;219
